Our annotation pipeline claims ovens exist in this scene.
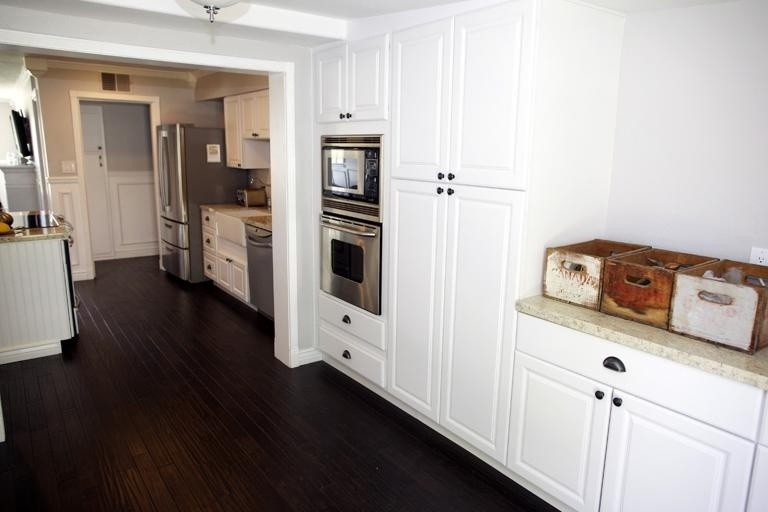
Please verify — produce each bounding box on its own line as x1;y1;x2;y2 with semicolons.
320;214;382;315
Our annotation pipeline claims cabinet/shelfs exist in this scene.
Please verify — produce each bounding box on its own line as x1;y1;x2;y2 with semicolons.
312;30;389;124
382;1;626;477
200;209;257;314
316;289;386;402
504;316;767;512
239;89;270;140
223;96;269;169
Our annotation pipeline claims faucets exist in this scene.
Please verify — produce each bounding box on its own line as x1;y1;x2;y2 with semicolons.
250;177;271;187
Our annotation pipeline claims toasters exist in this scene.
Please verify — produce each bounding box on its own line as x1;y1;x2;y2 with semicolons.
236;188;267;208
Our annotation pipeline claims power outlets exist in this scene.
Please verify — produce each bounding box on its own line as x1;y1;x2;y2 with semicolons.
748;246;768;266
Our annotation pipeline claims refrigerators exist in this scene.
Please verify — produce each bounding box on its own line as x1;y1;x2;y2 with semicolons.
156;122;251;284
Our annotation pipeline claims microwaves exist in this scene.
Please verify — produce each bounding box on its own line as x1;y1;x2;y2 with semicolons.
320;146;379;206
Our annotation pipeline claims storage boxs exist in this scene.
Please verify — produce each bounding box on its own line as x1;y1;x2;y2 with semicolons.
544;235;768;357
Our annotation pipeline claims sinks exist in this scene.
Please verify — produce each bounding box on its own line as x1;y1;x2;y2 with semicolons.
214;209;272;247
